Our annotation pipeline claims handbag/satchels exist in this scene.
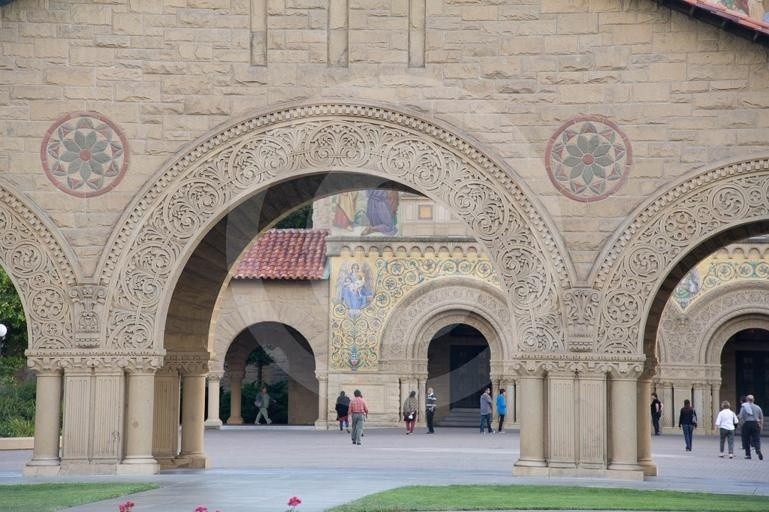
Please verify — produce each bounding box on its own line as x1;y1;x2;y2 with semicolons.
691;408;697;427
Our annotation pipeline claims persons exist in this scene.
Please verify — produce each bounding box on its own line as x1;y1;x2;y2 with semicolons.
678;400;697;452
650;392;664;434
479;387;495;434
348;389;368;445
738;394;763;461
253;385;277;424
346;263;365;293
402;389;418;434
331;190;399;236
341;276;360;309
713;400;739;458
335;391;351;433
426;387;437;434
495;388;506;433
734;394;755;450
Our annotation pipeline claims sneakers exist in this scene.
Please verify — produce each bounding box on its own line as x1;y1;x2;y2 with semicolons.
744;450;763;460
718;453;733;459
352;441;361;445
339;427;350;434
